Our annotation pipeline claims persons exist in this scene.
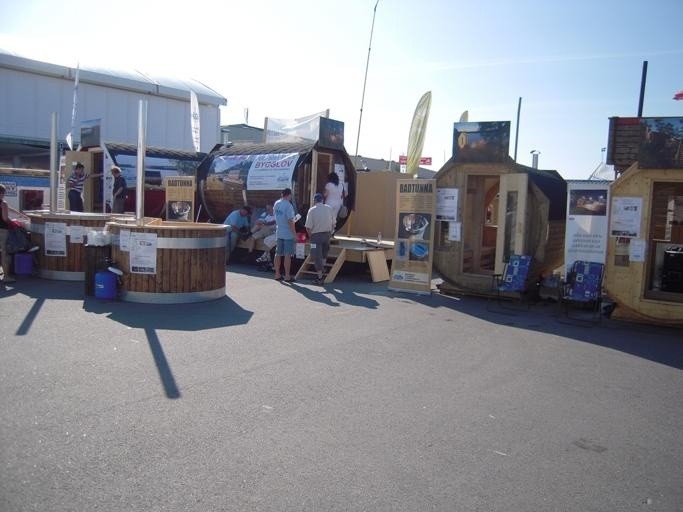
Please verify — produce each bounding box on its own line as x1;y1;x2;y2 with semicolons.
324;172;344;235
111;167;127;213
242;201;276;260
256;233;276;263
224;206;252;264
67;164;104;212
0;184;19;284
273;188;297;282
304;193;337;285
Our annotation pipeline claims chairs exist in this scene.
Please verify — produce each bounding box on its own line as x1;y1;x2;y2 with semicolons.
486;255;535;316
555;259;606;328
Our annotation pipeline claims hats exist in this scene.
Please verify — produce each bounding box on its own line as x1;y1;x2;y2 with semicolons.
313;194;322;201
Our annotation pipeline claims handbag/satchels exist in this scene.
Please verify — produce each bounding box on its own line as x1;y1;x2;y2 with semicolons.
6;221;32;253
338;205;348;219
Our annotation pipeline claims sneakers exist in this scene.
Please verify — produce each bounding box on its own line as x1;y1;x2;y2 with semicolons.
312;277;322;284
274;276;294;281
255;255;272;263
